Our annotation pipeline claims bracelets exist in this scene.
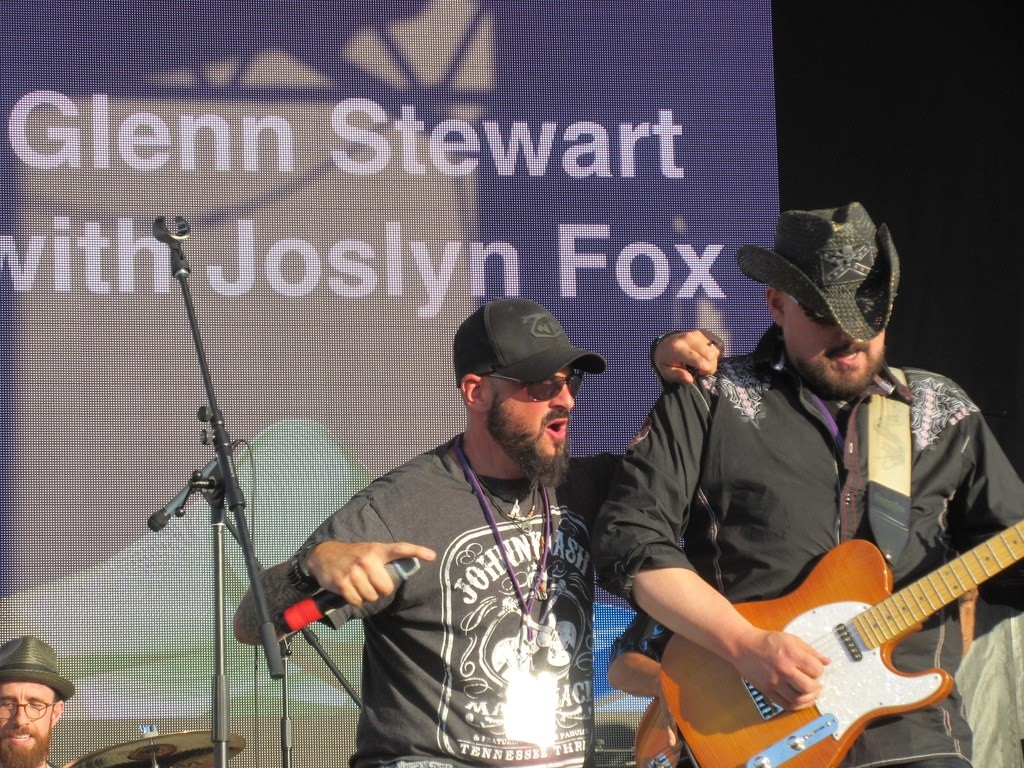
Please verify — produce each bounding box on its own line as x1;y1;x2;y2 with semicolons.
299;541;320;576
651;329;682;365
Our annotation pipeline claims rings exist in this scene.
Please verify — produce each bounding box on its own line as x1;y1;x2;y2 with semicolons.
706;340;716;346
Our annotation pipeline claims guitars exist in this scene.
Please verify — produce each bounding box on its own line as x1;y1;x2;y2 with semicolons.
656;519;1024;768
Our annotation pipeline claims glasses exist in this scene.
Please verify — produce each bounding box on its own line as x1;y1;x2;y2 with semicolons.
783;291;837;327
0;699;57;720
489;371;585;401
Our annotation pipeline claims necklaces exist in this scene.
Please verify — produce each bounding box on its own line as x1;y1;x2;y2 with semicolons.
465;455;549;601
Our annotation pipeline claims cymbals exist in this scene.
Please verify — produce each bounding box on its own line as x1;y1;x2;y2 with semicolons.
60;729;247;768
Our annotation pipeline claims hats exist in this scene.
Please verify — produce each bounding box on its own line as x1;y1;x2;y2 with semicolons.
736;202;901;343
452;299;607;387
0;635;75;702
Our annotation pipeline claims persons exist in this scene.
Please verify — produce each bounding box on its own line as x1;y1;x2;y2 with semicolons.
0;636;76;768
590;201;1023;768
233;299;726;768
606;613;701;768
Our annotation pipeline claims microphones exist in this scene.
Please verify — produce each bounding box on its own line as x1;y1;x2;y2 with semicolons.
148;439;241;532
274;557;421;642
